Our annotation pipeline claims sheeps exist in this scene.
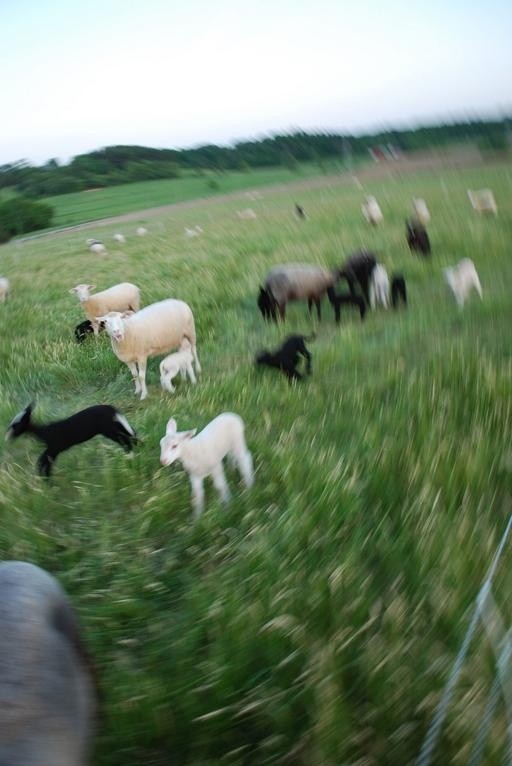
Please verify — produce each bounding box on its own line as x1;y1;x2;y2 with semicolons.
68;187;499;522
4;397;141;482
0;561;99;766
